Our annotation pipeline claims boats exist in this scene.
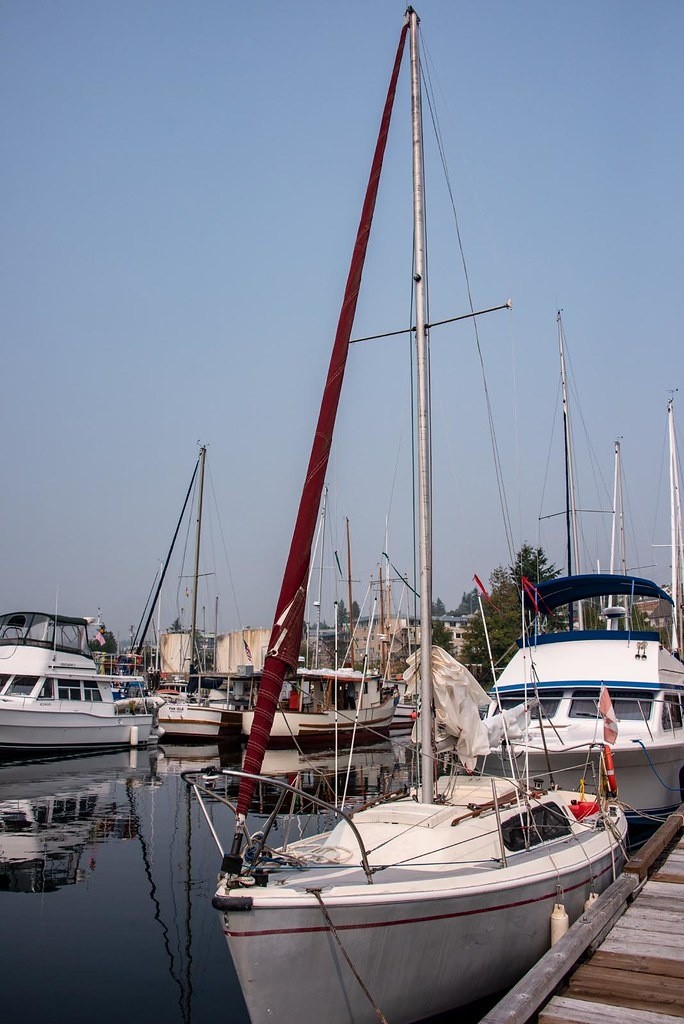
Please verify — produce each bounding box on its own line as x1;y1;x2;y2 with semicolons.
90;5;684;1024
1;586;166;749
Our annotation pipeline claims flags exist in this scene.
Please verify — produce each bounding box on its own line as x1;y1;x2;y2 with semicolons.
96;632;106;646
244;639;252;661
598;687;618;745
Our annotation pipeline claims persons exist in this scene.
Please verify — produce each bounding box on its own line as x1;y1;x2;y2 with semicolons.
667;646;679;661
343;684;350;709
252;682;257;704
289;683;299;711
541;612;566;634
